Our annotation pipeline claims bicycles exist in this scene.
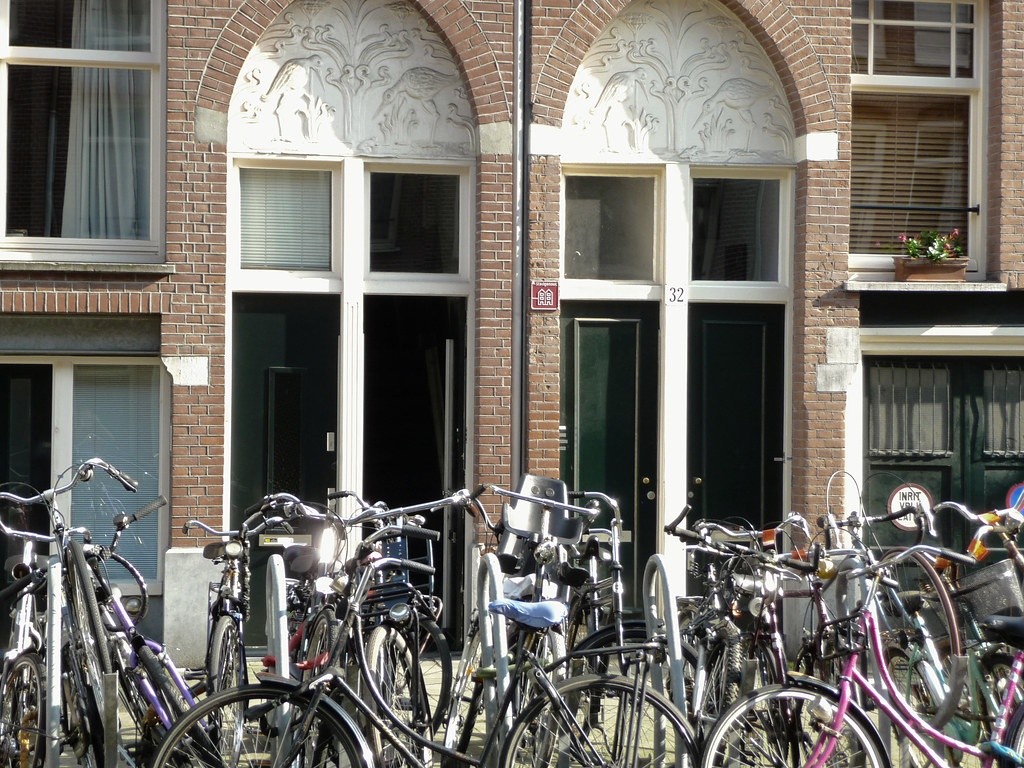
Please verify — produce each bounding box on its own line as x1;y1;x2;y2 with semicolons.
0;456;1023;767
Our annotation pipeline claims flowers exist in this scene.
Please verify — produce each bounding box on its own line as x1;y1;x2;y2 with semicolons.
897;228;964;263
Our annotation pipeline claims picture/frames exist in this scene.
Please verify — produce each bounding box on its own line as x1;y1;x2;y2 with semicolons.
892;255;970;283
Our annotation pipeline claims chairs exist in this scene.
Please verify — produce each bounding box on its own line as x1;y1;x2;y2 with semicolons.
497;473;586;547
